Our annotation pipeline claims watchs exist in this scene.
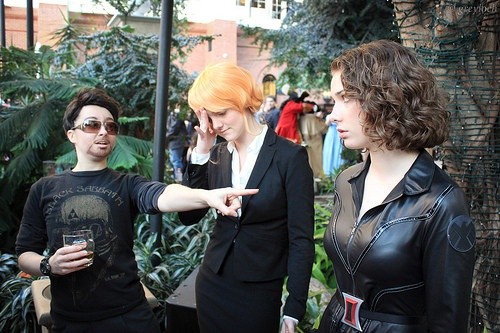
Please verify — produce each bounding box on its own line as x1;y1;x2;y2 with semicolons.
40;254;55;277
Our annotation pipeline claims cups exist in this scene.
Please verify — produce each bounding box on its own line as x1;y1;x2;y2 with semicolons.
62;229;94;267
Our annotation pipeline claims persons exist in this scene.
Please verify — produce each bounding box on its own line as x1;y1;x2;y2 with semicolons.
178;61;316;333
318;39;476;333
14;86;259;333
254;89;358;177
166;104;197;183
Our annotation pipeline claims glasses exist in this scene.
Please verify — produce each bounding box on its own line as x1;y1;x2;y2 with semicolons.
71;120;120;135
